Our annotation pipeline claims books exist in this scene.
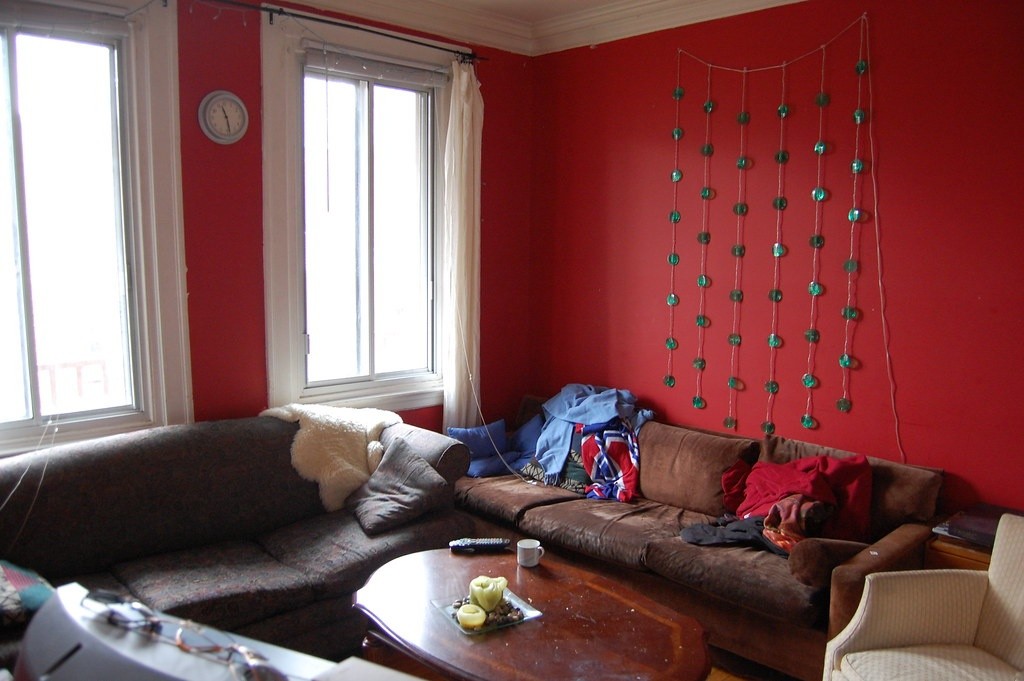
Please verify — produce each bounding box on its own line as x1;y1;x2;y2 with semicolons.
932;501;1024;548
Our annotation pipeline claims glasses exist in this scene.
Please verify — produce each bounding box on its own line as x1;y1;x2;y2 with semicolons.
78;587;162;639
177;620;287;681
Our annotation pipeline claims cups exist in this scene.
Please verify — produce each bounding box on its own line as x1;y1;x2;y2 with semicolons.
516;539;545;567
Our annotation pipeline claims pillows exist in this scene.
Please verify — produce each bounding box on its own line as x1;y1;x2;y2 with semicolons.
787;538;873;586
448;418;521;479
503;414;544;474
345;436;448;535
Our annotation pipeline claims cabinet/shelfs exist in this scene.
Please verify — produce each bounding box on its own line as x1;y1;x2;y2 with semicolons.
924;534;992;571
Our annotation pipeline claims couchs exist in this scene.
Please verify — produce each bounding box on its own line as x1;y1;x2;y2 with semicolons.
0;417;476;669
823;514;1024;681
453;398;943;681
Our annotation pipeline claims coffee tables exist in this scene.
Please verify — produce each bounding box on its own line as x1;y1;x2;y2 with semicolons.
351;546;711;681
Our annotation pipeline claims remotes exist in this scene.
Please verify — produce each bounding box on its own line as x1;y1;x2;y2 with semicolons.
449;538;510;551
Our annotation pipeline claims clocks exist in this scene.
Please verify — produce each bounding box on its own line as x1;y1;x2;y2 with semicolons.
198;90;249;145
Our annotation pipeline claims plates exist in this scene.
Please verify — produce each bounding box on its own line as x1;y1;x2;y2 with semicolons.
430;585;543;636
